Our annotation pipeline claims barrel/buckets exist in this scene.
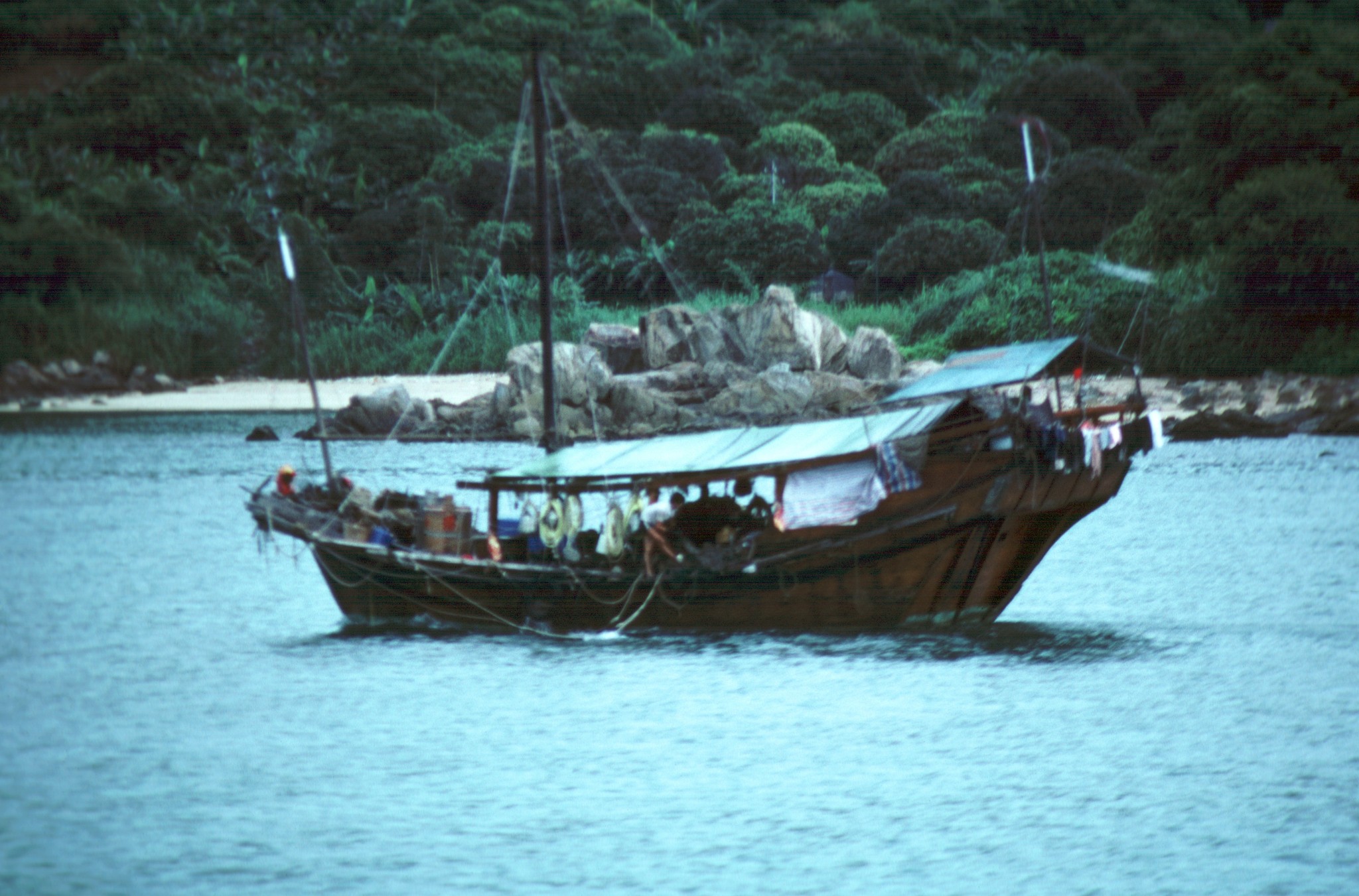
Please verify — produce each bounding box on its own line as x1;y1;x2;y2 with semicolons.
367;527;394;549
496;520;520;540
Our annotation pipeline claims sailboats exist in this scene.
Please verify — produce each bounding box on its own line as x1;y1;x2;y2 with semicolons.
240;16;1157;668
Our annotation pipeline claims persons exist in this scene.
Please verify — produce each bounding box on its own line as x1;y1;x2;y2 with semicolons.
622;484;685;580
